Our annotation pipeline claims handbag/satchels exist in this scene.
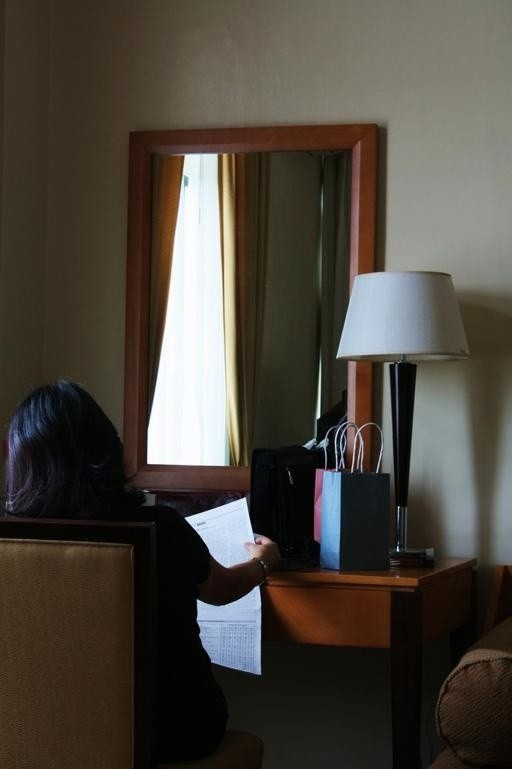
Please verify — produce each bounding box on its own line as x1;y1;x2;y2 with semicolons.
314;422;390;572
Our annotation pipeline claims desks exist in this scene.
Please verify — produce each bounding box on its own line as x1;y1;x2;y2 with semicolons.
260;556;477;769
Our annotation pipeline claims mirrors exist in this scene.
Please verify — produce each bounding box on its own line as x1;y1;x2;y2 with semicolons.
124;123;378;491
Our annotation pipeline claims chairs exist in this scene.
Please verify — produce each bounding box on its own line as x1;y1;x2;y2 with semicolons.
0;519;264;768
429;565;511;769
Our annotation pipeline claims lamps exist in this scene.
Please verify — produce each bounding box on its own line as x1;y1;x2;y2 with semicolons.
335;272;470;564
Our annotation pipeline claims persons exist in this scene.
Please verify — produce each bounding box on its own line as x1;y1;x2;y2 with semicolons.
0;381;282;769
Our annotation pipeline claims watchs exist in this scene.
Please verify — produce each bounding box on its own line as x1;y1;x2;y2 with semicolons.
249;556;270;587
